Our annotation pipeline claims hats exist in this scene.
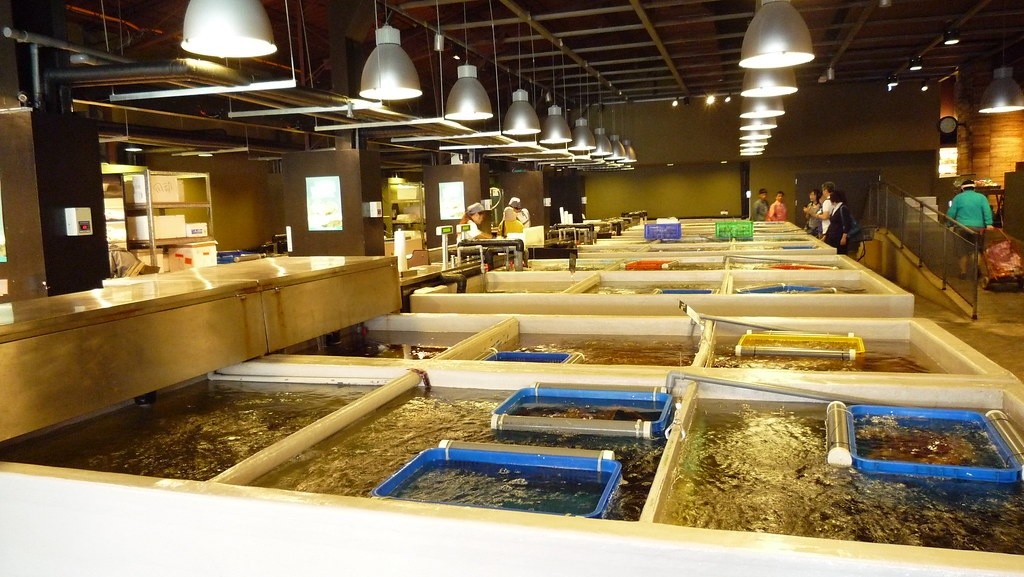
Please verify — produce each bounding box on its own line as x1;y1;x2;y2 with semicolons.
508;197;521;208
467;203;485;213
503;207;516;222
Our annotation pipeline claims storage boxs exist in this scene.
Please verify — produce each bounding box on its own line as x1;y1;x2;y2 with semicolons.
0;213;1024;577
100;172;216;267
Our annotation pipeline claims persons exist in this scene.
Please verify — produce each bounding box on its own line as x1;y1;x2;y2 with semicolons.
947;180;994;279
802;182;853;255
508;197;531;228
497;207;524;238
753;188;771;221
767;191;787;222
456;203;493;245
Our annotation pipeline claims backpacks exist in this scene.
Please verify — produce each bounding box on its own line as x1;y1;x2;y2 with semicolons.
840;205;860;252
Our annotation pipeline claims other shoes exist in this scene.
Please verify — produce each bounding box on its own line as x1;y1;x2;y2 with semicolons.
959;272;967;280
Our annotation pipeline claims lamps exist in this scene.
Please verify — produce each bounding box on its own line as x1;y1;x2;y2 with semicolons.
672;85;733;108
589;82;612;155
616;96;637;163
921;80;929;92
739;0;816;158
568;63;596;150
944;23;960;45
503;14;541;134
540;40;572;144
908;57;925;71
181;0;279;58
358;0;423;99
979;21;1024;114
886;76;898;87
444;0;492;121
604;88;626;160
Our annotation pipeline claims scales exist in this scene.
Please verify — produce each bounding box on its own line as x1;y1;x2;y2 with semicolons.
431;224;470;267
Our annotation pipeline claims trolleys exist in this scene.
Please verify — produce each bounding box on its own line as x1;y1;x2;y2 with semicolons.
977;227;1024;290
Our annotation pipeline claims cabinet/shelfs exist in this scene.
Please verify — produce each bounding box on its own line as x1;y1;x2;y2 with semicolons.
125;174;212;275
